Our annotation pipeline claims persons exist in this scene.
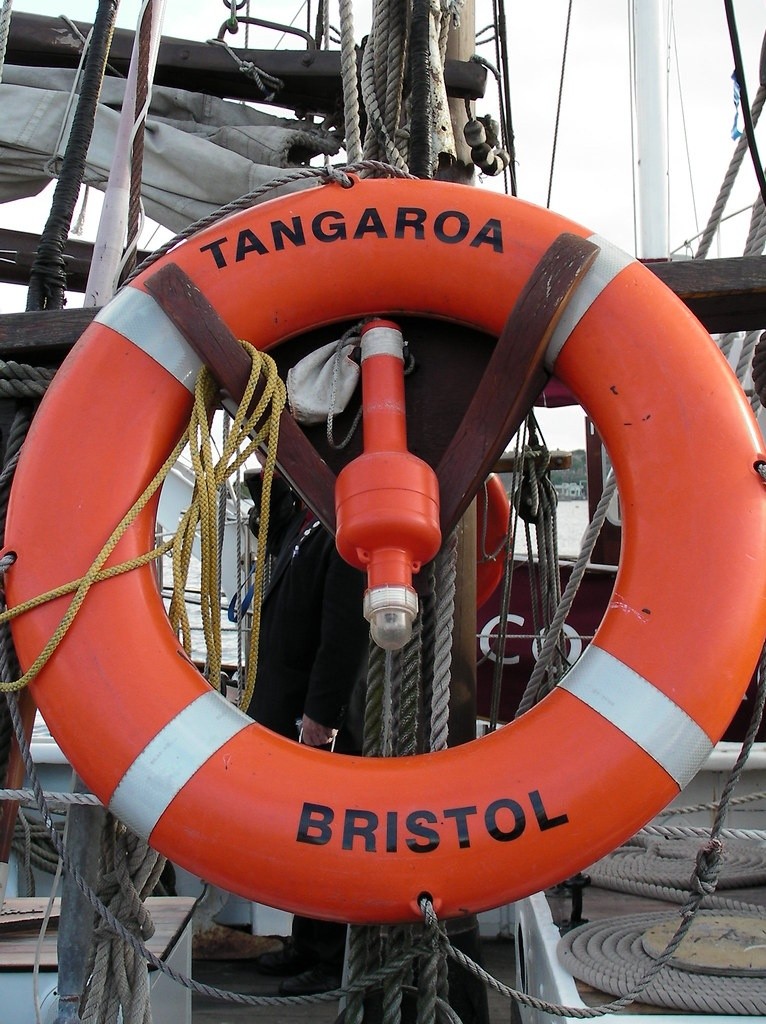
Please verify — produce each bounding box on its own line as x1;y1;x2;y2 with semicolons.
243;449;361;997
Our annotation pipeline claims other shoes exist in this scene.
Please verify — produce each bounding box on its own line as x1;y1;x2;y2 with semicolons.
257;939;309;975
278;974;342;997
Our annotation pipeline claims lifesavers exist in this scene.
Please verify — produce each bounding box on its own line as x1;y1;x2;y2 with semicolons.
1;173;766;925
